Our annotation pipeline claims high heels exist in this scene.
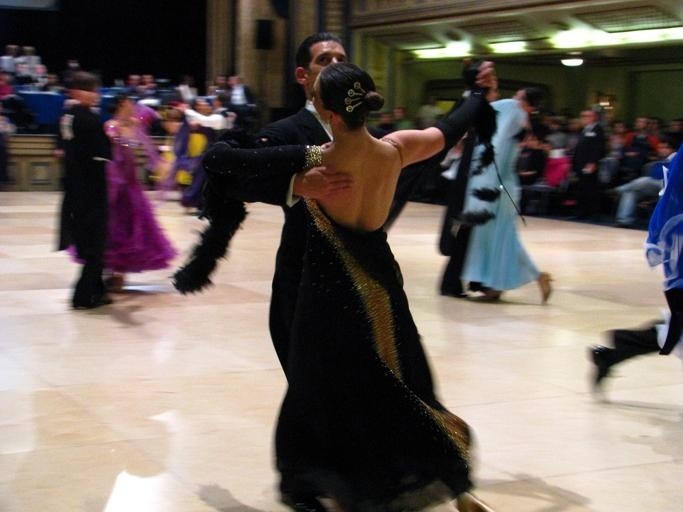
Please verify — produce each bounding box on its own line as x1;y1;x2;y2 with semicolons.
537;272;557;305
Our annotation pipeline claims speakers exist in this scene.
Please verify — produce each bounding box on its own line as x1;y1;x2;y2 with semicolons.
254;19;277;50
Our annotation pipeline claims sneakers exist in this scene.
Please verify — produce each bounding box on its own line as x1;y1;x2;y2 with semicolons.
440;283;504;304
590;343;610;400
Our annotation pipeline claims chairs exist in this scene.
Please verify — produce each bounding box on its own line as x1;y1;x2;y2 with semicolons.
2;67;221;137
